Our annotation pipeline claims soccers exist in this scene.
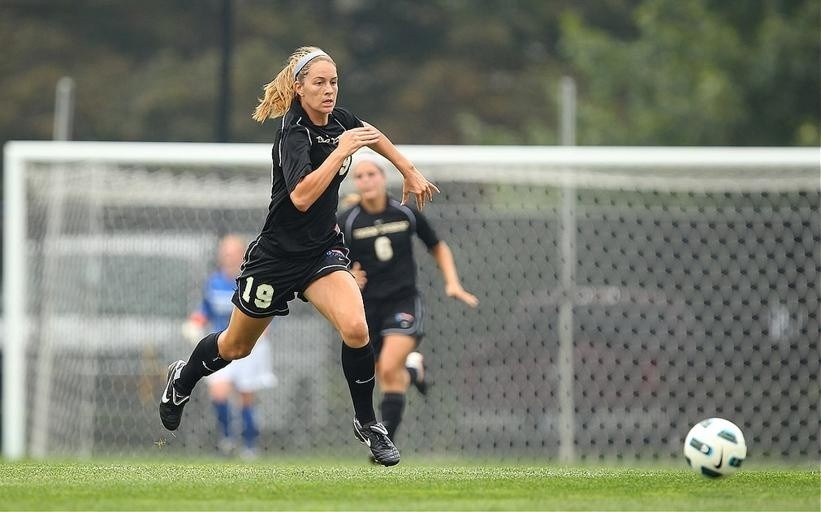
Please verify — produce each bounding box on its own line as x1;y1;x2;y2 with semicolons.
683;418;746;480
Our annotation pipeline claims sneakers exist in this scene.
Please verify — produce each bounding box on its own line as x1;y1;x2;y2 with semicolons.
407;353;427;395
351;417;400;466
159;359;190;431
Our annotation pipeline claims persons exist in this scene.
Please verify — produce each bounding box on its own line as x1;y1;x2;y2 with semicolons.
158;45;440;466
183;230;280;462
337;155;480;463
